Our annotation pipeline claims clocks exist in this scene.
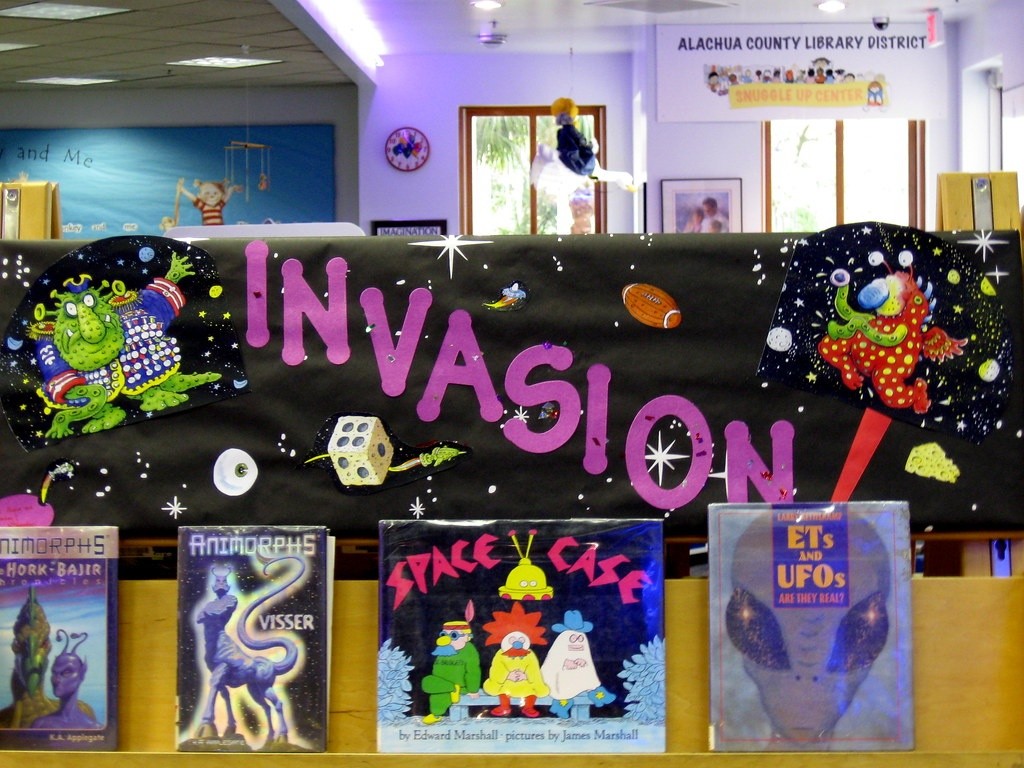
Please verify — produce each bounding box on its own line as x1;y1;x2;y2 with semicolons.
385;127;431;173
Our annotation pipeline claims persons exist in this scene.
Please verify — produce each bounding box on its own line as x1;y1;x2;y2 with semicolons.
529;97;635;198
683;197;730;234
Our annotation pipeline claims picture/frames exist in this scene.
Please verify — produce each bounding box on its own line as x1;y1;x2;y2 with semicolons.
370;219;448;237
660;177;744;234
